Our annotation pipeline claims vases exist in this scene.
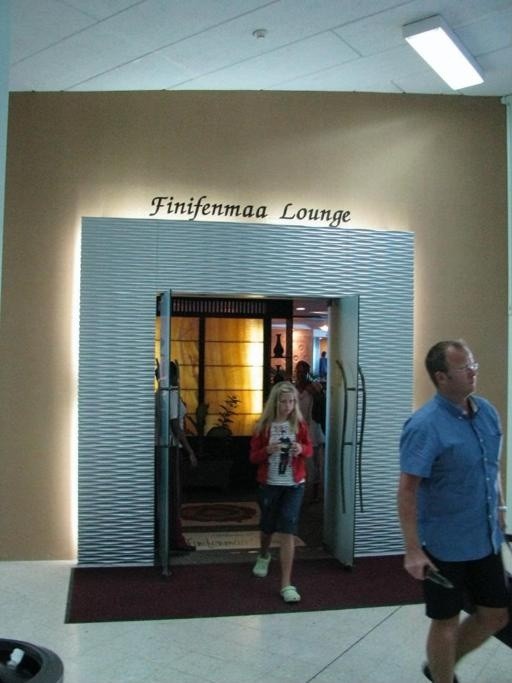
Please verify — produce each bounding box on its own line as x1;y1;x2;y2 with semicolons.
273;333;284;357
273;364;284;384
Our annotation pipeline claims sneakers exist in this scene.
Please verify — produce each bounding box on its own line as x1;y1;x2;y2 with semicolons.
169;535;196;555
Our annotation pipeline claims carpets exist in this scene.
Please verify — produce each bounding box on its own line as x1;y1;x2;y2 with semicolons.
176;500;265;526
183;529;308;551
62;551;511;649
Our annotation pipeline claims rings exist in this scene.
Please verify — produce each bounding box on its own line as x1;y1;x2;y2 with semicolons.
294;448;297;451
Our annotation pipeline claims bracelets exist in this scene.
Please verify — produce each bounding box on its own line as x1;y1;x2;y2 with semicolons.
497;503;509;513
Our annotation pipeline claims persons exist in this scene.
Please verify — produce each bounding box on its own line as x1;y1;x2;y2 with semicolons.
247;380;316;606
155;358;198;555
318;350;327;378
393;337;511;683
292;358;327;505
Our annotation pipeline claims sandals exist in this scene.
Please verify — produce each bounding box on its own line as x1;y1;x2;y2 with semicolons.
280;586;301;603
252;552;271;578
